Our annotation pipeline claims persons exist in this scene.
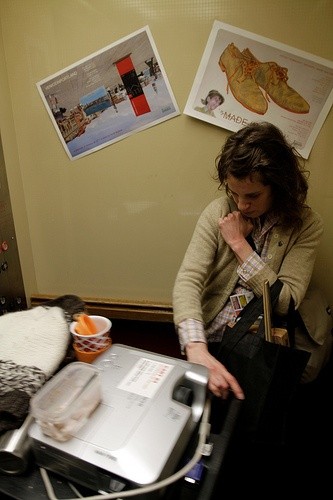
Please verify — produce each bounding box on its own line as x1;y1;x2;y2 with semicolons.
171;122;331;500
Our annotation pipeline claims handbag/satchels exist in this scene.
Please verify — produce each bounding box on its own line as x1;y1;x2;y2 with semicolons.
207;278;311;432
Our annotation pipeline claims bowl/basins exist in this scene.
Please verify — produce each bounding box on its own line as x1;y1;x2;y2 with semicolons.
73;343;111;363
70;316;112;351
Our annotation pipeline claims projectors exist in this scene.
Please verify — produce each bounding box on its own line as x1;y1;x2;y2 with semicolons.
27;343;211;500
